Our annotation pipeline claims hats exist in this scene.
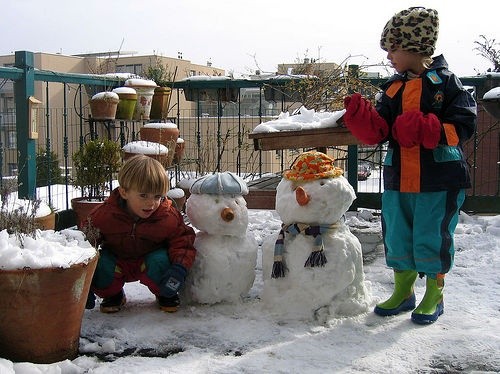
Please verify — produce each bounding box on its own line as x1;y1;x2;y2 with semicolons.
379;7;439;56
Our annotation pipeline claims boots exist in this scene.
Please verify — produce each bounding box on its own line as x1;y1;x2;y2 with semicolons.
411;276;444;324
374;269;418;317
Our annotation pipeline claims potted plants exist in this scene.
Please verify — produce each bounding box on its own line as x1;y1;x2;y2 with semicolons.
69;135;124;232
142;61;174;119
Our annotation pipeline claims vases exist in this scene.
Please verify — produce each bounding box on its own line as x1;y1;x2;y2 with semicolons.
116;93;138;121
123;150;166;165
0;252;100;365
125;85;156;119
89;99;118;120
141;127;179;170
32;213;55;231
172;142;186;164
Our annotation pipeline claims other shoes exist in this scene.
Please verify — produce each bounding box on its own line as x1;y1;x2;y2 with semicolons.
156;294;180;312
100;287;127;312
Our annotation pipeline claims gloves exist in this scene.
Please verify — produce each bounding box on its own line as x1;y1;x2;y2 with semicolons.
157;262;190;308
85;289;96;309
392;111;441;150
343;93;389;145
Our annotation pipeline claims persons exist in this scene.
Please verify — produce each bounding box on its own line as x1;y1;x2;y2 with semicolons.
79;153;197;313
343;6;478;326
